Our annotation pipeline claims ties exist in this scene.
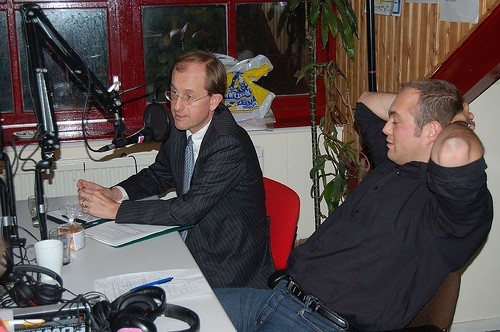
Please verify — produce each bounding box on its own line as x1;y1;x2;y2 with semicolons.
178;135;194;242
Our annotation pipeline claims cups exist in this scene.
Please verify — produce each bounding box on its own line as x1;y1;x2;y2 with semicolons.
59;223;87;250
48;229;71;264
28;194;49;227
34;240;64;281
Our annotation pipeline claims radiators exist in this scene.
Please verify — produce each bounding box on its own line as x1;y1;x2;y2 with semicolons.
6;143;267;200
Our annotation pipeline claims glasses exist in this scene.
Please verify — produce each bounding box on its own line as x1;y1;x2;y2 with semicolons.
165;89;208;106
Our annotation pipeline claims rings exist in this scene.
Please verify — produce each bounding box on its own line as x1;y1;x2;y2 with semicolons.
82;199;86;207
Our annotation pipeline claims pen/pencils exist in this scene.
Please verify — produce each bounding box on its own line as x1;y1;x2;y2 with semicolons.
131;276;174;291
61;214;76;221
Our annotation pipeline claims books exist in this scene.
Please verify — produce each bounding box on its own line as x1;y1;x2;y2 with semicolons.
47;196;195;249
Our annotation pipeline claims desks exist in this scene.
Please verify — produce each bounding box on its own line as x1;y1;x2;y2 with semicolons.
15;192;237;332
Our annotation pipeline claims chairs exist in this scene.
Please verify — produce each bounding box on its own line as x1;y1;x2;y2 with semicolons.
389;268;461;332
263;175;300;290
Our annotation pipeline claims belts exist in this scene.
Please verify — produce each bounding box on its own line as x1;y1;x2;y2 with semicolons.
284;276;355;332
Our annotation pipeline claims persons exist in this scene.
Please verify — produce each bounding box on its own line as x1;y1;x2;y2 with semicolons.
212;80;494;332
77;52;275;290
